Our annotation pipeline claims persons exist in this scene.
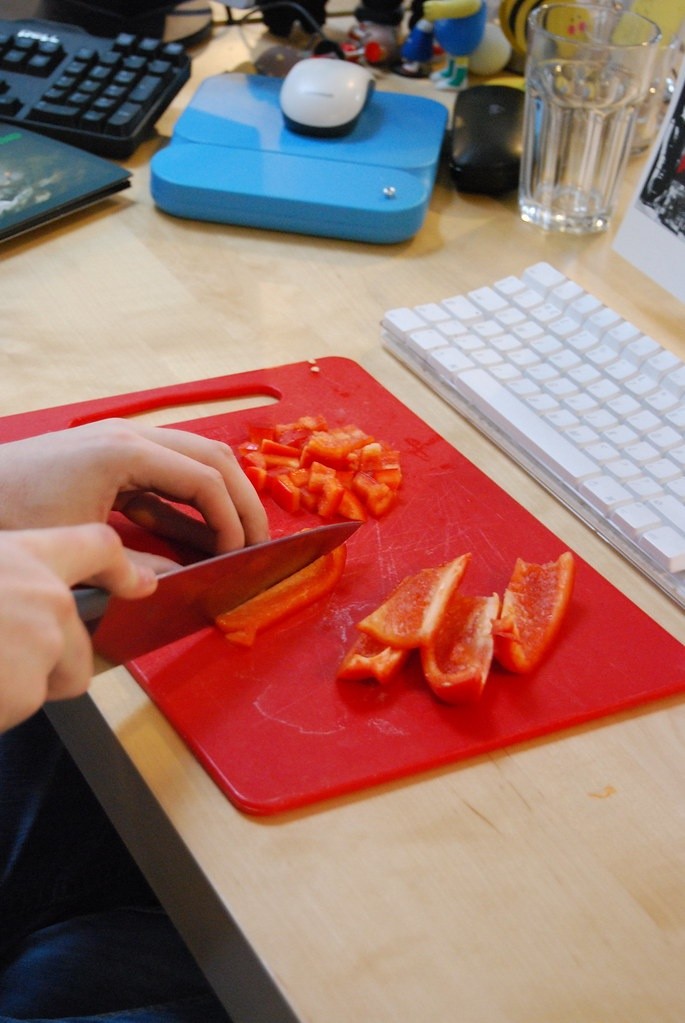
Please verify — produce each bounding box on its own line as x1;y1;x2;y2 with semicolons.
0;412;269;736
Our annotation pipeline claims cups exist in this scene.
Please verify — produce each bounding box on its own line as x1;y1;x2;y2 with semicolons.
519;3;685;235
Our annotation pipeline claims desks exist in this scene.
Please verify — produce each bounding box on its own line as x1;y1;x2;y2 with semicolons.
0;0;684;1023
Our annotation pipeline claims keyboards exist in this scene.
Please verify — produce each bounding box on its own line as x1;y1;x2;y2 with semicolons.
378;260;685;610
0;17;192;162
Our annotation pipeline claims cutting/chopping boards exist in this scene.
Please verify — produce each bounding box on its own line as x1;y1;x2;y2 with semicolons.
0;355;685;814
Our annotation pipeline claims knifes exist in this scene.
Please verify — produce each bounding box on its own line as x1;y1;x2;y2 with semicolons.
70;521;373;666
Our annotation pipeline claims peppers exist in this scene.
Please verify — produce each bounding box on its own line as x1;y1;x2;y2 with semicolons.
212;528;348;642
237;415;404;524
335;553;574;703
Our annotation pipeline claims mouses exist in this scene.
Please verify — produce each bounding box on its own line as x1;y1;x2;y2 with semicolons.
280;59;375;139
